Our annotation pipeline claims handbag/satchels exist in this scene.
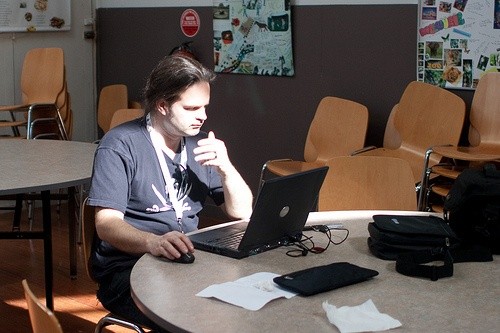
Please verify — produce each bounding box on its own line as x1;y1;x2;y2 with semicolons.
368;215;492;281
443;161;500;255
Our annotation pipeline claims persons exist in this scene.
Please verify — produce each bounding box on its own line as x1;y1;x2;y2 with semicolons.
87;53;253;333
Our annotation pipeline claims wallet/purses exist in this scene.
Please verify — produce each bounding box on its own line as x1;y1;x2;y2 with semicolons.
273;262;379;297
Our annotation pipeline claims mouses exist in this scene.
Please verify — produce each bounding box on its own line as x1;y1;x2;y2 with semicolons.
155;244;195;263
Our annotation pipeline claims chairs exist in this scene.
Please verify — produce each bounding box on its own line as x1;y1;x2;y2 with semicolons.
256;73;500;211
0;46;145;333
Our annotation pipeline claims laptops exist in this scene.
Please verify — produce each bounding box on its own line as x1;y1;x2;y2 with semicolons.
189;166;330;258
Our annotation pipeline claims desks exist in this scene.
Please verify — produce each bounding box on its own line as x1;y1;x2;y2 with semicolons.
131;211;500;333
0;139;98;311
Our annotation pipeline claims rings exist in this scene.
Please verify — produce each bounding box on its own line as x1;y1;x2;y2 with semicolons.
214;151;217;158
